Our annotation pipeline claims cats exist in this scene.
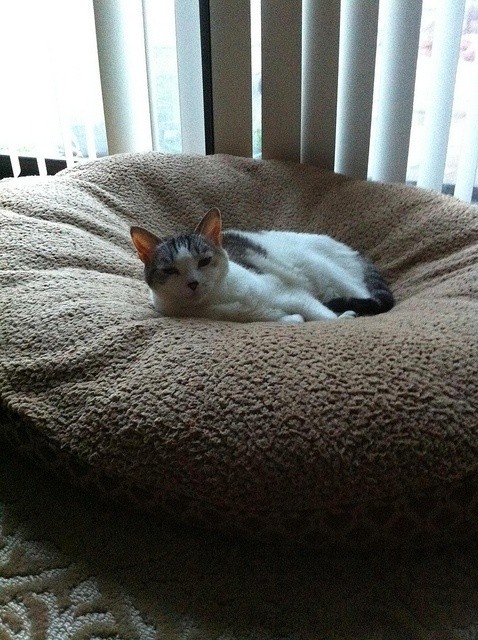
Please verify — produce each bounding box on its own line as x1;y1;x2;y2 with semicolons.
129;207;394;324
